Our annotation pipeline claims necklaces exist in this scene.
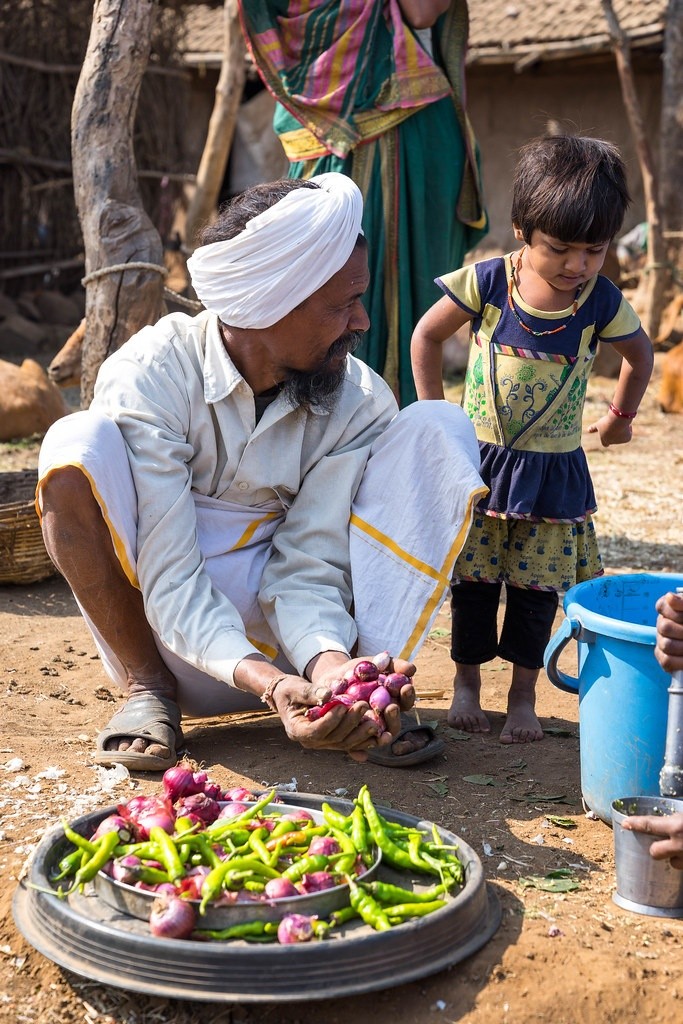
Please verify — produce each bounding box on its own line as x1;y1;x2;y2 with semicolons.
508;244;583;337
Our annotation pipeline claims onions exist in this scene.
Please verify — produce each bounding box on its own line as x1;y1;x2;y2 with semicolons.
303;650;412;740
89;756;370;944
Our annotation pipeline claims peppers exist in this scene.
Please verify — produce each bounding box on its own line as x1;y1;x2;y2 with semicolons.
49;784;467;942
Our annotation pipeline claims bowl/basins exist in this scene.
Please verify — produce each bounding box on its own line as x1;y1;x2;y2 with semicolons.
608;796;683;917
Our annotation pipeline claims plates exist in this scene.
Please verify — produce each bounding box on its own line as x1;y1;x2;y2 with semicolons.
87;801;382;930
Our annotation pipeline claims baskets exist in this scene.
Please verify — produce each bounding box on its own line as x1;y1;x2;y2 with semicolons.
0;470;60;586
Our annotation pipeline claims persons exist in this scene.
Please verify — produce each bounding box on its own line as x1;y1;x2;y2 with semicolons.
409;131;654;745
34;172;491;770
240;0;489;412
623;592;683;859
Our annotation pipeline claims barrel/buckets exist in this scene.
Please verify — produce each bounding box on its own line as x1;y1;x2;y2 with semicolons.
544;573;683;827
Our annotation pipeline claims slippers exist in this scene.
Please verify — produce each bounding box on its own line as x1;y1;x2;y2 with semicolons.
366;711;447;768
94;696;181;769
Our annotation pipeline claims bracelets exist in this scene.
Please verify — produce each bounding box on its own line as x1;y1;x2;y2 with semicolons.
259;674;296;714
610;404;637;418
